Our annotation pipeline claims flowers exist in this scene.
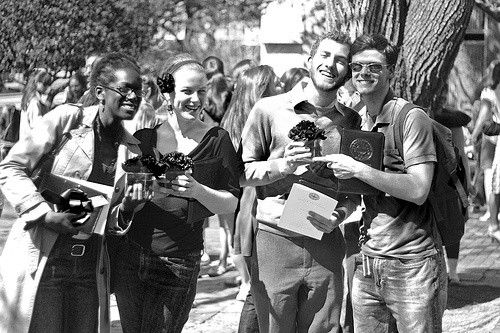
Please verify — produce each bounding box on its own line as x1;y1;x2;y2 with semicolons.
288;120;327;141
156;72;175;94
120;151;194;173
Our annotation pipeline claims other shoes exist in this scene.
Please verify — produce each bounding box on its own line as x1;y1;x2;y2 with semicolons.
208;266;226;277
479;211;491;222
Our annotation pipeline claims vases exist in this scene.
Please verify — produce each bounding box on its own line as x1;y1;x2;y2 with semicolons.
295;140;321;158
124;171;191;198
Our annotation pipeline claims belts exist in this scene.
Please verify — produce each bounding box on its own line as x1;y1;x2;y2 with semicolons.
53;244;91;256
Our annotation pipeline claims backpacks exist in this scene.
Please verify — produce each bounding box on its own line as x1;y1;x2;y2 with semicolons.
394;103;472;245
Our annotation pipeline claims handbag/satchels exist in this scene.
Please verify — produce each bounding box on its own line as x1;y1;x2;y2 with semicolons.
30;103;84;190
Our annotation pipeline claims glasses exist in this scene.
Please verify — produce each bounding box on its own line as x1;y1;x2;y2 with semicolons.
349;62;390;73
106;86;147;97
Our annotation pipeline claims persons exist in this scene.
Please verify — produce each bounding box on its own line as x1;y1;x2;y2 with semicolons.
107;53;242;333
0;57;500;284
237;31;362;333
220;65;285;333
312;34;447;333
0;52;153;333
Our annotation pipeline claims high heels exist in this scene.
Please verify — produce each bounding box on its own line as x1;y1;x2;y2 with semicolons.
488;225;500;244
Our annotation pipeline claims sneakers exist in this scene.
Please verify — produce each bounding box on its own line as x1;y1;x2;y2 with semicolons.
448;275;461;286
236;283;251;301
